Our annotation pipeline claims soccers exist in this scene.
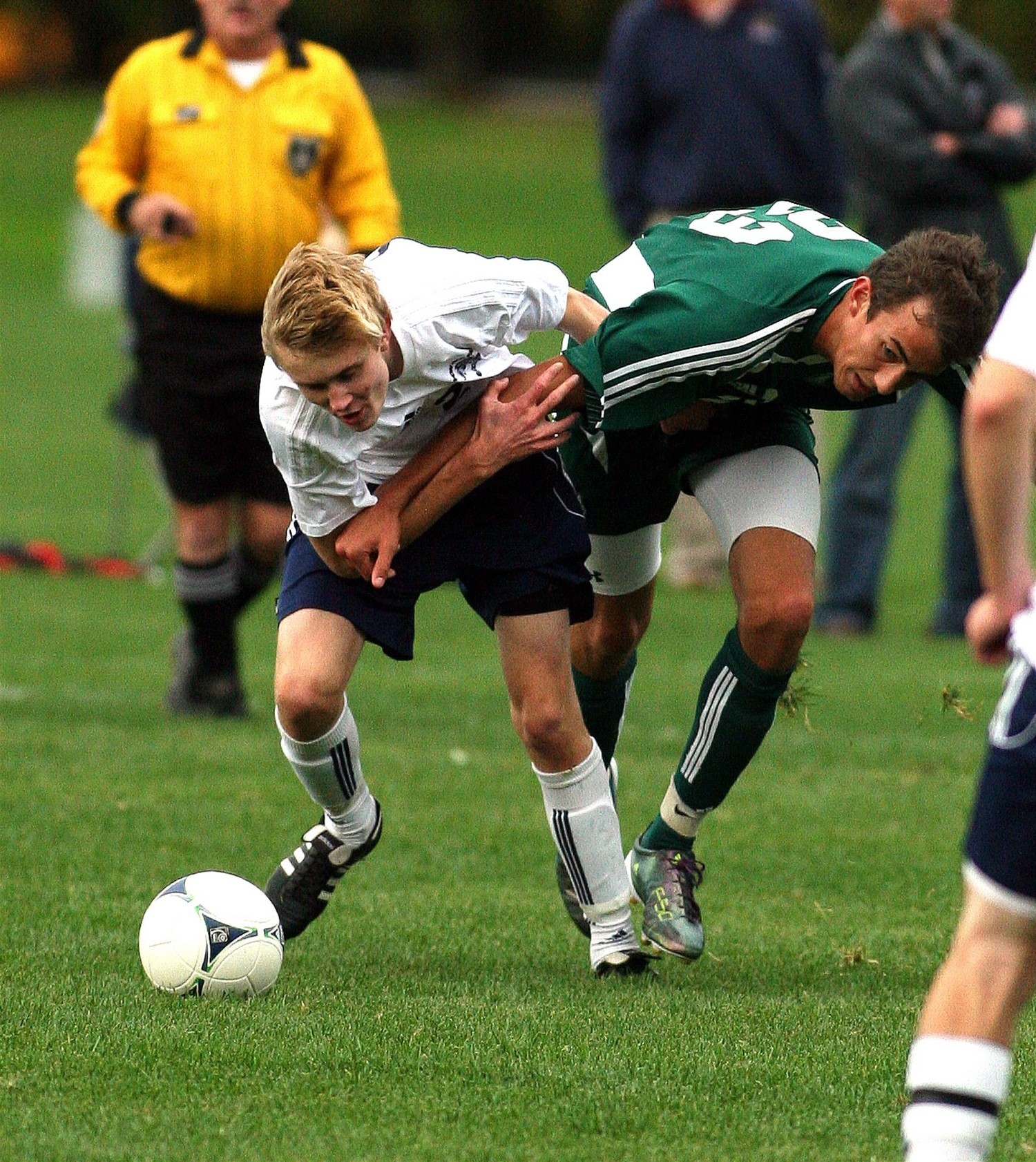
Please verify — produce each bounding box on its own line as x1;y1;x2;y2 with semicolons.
138;870;285;998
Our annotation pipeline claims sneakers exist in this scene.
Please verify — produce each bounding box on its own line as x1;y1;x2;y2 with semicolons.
556;757;618;941
596;953;660;980
263;798;382;940
624;822;705;963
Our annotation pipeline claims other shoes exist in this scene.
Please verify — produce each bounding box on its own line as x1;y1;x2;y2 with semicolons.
211;682;251;721
159;632;206;717
818;620;859;637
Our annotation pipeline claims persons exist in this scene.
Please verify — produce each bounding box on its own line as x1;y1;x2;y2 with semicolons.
77;0;403;717
825;0;1036;642
336;198;1002;966
66;0;198;441
900;242;1036;1162
597;0;844;586
258;235;647;978
